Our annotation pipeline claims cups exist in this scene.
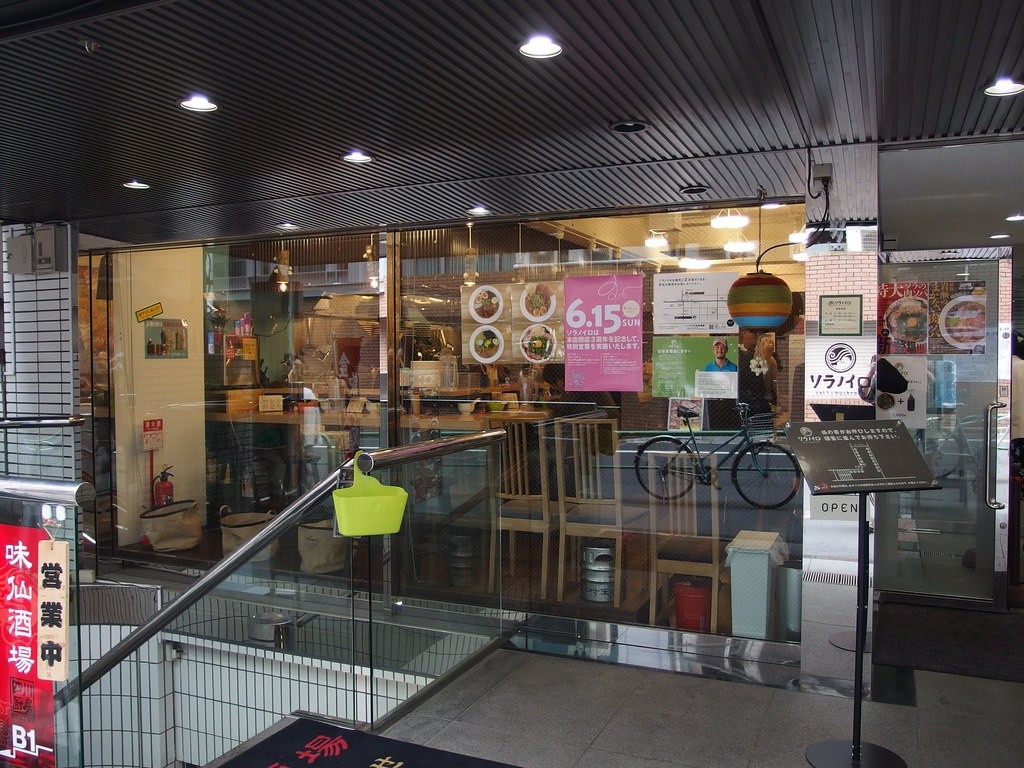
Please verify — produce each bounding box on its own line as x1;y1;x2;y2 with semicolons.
501;393;518;409
492;391;502;401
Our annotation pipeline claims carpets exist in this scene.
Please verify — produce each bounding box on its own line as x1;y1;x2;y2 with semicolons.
219;718;523;768
873;602;1024;683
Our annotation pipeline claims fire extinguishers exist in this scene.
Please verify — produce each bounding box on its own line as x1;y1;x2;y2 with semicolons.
152;465;174;508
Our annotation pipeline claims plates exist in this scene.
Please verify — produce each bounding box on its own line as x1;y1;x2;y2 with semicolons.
469;286;504;324
469;325;504;363
883;296;927;345
939;295;986;350
520;324;557;363
520;284;556;322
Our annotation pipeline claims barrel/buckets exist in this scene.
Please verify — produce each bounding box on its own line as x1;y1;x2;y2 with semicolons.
670;575;712;631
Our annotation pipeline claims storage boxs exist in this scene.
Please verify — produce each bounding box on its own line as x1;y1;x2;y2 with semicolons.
410;360;441;389
399;368;412;387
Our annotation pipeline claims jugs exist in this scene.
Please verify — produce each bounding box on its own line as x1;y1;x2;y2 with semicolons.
437;353;458;391
518;375;538;411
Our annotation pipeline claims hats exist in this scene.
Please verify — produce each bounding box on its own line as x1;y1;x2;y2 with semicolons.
712;338;728;347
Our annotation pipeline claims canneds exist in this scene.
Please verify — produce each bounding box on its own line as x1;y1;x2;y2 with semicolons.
151;343;170;356
234;318;250;335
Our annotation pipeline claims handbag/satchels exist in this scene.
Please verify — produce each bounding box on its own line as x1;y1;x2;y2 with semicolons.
140;499;202;552
219;503;279;562
297;518;346;574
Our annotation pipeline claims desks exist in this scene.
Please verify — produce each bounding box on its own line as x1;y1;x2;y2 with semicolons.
207;410;553;432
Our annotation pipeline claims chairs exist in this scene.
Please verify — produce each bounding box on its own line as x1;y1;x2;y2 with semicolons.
479;412;577;600
552;416;650;609
898;518;926;580
646;448;727;634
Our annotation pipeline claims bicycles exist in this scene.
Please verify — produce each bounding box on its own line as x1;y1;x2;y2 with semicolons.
634;403;801;510
912;400;984;480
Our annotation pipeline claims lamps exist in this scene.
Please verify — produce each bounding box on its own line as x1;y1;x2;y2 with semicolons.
274;241;291;293
722;232;756;252
365;234;379;288
711;208;749;228
644;228;670;247
788;224;807;262
555;231;565;280
463;224;477;285
513;223;526;284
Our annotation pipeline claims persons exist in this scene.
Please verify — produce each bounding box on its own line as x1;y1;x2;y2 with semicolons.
705;338;737;372
536;364;598;502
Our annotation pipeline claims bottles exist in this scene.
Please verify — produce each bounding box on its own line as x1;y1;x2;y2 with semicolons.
481;365;512;387
148;328;186;355
205;303;254;358
532;364;564;407
288;401;298;412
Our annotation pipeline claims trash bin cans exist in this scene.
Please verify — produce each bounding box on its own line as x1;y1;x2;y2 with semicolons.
444;533;478;590
775;559;801;634
582;540;628;605
246;612;295;650
729;529;780;640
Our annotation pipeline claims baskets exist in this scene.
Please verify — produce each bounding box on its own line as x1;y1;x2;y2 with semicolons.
747;412;778;443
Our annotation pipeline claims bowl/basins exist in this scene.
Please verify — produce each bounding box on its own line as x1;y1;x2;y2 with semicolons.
458;403;475;414
487;402;505;411
319;400;331;413
298;401;319;412
366;403;381;414
